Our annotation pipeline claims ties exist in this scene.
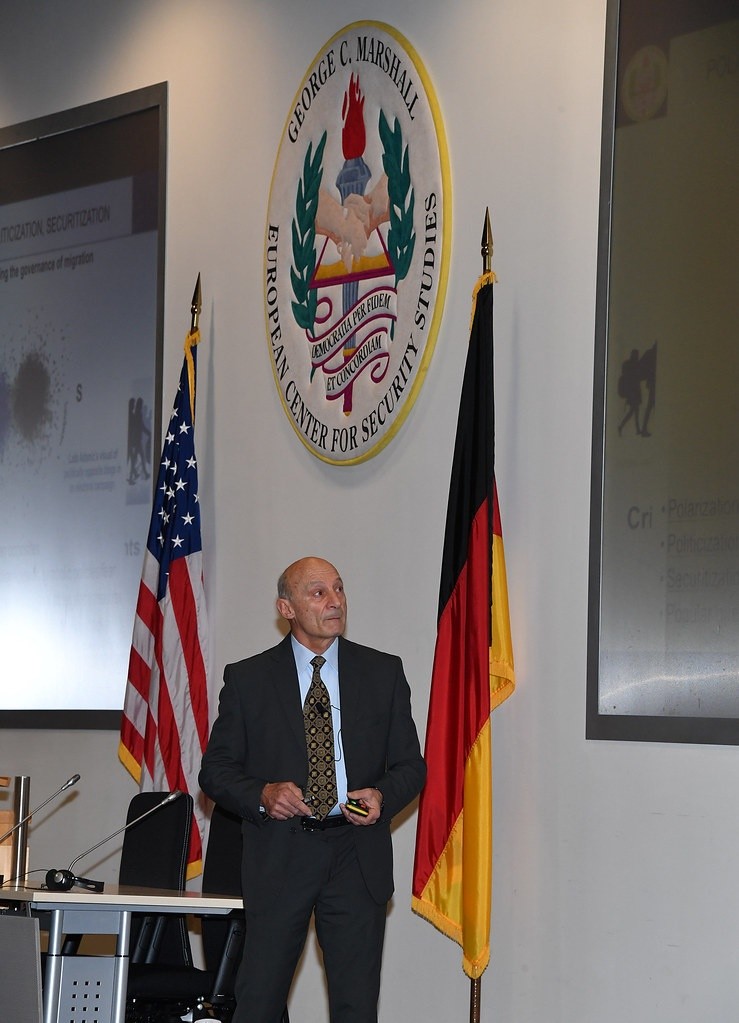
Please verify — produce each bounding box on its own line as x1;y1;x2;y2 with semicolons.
303;656;338;820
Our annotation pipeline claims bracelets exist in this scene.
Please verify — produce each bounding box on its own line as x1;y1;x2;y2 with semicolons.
375;788;385;808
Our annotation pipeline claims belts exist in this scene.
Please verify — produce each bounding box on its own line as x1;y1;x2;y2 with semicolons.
301;815;349;832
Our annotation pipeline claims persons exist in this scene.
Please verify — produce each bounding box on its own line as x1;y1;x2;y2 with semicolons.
198;556;426;1023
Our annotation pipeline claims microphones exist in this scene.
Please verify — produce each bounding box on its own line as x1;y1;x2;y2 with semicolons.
0;774;81;840
68;788;183;871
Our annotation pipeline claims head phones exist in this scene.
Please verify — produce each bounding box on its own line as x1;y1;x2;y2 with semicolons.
45;869;104;893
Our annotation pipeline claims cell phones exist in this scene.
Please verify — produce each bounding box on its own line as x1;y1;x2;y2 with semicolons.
346;799;369;817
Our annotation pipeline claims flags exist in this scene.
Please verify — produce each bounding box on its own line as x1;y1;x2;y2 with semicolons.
117;329;216;882
411;272;516;980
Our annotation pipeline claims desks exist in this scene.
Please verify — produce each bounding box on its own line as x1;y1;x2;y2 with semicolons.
0;866;243;1023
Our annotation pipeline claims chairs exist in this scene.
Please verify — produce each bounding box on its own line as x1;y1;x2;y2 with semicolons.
59;790;246;1023
